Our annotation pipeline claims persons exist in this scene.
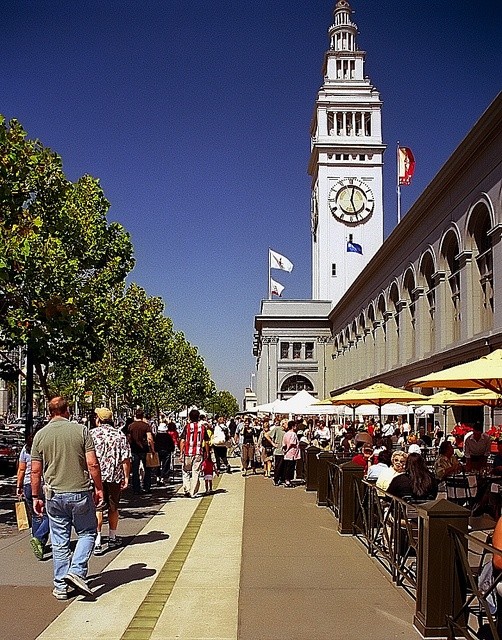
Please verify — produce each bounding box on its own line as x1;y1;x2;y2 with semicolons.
93;529;169;555
67;561;156;602
28;396;103;600
88;405;130;554
15;420;51;562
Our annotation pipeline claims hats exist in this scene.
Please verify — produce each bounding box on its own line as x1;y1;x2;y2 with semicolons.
95;407;112;421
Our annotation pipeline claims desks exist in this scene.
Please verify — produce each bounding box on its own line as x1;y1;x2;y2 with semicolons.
468;515;495;532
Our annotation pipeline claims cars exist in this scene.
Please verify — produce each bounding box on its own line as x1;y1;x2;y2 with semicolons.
8;417;39;428
0;430;24;467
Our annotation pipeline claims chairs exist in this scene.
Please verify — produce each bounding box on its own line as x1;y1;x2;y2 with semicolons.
443;470;473;507
425;453;436;472
397;495;436;544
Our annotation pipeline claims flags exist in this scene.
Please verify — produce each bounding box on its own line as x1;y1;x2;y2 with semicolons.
345;239;365;256
396;145;416;187
266;244;295;274
268;277;287;299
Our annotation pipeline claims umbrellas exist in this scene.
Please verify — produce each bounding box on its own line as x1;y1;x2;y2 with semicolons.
240;382;502;422
409;345;502;387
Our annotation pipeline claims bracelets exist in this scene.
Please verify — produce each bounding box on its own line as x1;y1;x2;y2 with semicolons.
16;485;23;488
31;493;44;498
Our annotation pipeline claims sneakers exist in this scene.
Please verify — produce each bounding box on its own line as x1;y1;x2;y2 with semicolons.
93;546;101;555
52;586;67;600
64;573;94;597
27;537;47;561
109;536;123;547
